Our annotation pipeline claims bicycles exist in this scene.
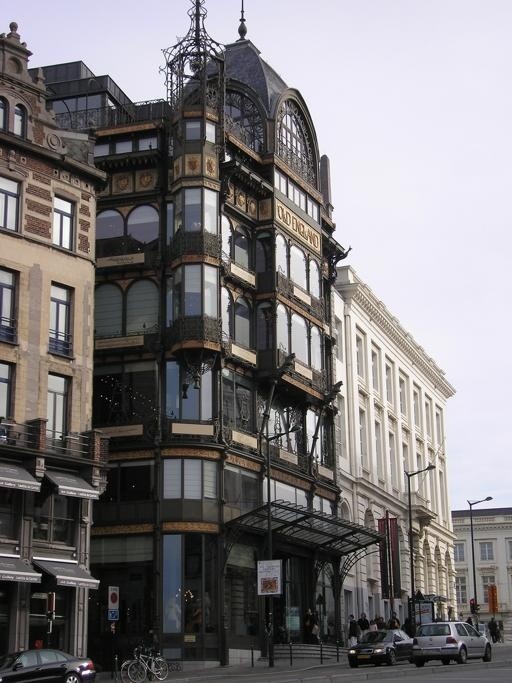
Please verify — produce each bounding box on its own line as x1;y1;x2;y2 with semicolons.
120;639;169;682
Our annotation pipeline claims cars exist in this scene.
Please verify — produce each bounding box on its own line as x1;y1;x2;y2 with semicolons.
348;629;414;667
1;646;96;683
411;620;491;666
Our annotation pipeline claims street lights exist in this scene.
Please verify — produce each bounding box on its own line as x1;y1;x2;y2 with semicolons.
466;496;494;630
266;424;303;666
405;462;436;635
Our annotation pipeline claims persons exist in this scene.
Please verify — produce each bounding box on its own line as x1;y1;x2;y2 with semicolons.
497;620;505;643
368;619;378;631
356;611;370;643
408;619;416;636
374;618;380;627
487;617;498;642
387;612;400;629
401;618;411;637
465;616;474;626
347;614;361;649
303;607;316;643
375;617;386;629
